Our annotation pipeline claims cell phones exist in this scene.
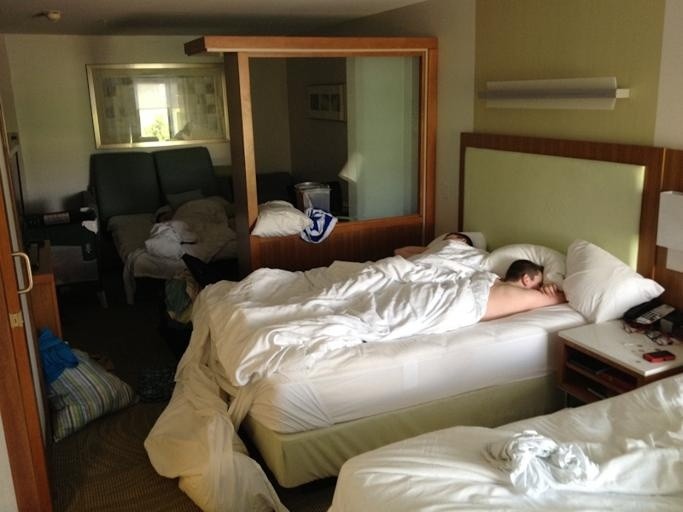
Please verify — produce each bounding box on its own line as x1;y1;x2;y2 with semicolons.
643;350;675;362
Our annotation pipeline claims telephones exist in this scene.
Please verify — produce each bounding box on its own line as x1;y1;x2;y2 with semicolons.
623;298;678;330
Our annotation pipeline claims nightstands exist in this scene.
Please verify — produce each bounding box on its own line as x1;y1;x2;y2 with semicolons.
552;319;683;409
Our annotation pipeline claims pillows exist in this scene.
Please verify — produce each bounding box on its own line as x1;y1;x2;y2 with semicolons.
481;241;564;291
564;238;665;325
426;230;487;250
250;199;313;238
166;188;204;211
153;204;172;217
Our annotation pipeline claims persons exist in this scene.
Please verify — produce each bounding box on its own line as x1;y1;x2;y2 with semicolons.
396;233;473;260
481;259;567;323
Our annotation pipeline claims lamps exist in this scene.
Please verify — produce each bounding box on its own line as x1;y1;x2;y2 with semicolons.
337;152;363;183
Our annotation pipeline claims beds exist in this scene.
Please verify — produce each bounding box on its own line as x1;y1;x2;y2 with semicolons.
330;372;683;510
89;146;236;274
200;130;671;488
